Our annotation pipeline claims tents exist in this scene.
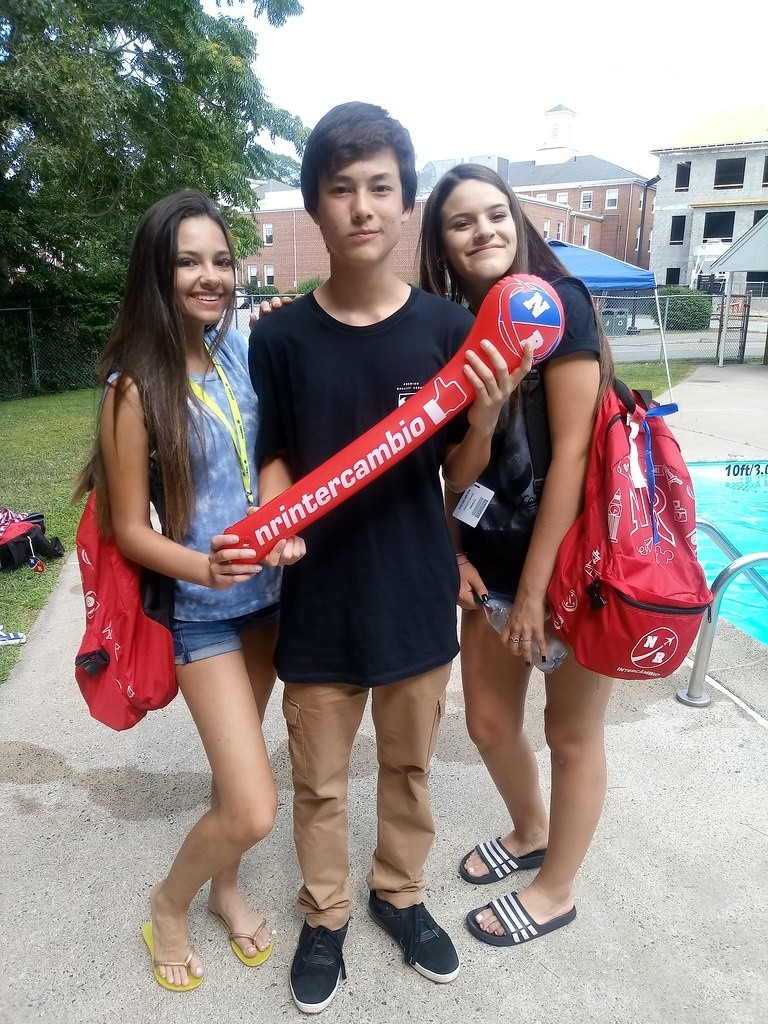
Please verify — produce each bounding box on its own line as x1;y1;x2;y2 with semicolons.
543;237;673;403
708;210;768;368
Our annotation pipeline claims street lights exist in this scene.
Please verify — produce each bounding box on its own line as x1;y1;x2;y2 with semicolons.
627;173;662;335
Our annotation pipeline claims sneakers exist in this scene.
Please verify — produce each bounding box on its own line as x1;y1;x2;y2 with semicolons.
366;887;461;984
288;915;350;1014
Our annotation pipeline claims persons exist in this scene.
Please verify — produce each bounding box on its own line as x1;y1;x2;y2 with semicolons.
249;96;538;1018
414;165;621;953
98;189;293;997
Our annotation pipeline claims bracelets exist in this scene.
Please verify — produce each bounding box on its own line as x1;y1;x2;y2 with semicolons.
456;552;468;557
457;560;470;566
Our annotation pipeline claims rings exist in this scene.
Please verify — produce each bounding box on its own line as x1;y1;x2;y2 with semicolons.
521;636;531;642
509;635;520;643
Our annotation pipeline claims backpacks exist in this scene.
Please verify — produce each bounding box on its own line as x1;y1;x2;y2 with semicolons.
74;372;177;733
545;378;717;681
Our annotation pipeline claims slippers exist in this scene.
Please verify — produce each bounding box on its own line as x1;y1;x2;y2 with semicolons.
458;837;548;883
210;909;274;968
143;922;204;992
466;889;577;947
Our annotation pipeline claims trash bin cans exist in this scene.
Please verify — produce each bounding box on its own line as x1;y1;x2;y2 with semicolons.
600;306;629;335
